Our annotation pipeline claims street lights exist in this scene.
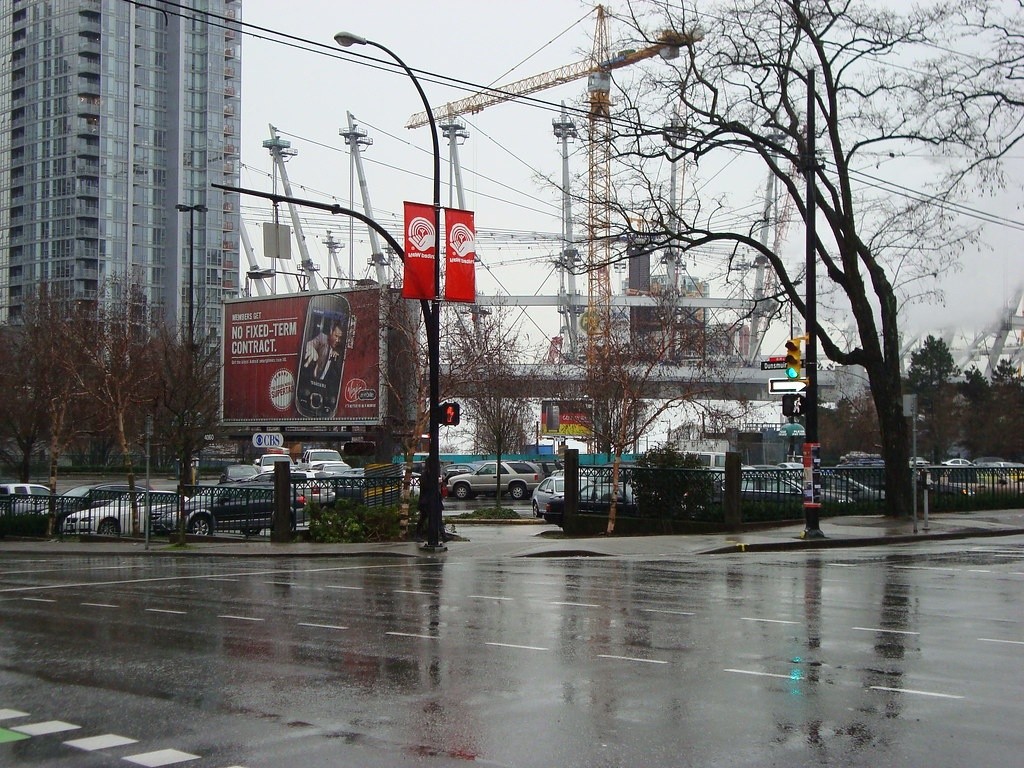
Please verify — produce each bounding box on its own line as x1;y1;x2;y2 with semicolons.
175;204;208;483
333;31;441;547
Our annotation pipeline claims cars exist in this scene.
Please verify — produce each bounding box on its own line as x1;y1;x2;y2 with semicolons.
532;476;595;518
0;484;50;514
544;483;635;526
25;483;153;515
820;473;885;501
909;456;1024;495
220;465;258;482
61;491;190;535
253;449;364;486
445;463;486;473
751;462;804;480
715;474;855;504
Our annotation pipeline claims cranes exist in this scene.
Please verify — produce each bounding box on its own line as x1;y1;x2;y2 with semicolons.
402;4;707;454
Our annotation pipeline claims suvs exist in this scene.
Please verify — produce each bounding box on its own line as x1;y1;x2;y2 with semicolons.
446;462;544;501
243;469;336;503
150;480;305;536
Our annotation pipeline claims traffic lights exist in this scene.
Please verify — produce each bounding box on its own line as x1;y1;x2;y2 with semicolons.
442;402;460;426
783;394;806;416
785;340;799;378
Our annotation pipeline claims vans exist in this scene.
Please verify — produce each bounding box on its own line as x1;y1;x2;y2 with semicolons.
675;451;758;482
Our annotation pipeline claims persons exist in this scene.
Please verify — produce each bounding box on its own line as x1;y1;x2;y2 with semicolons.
303;320;344;382
413;455;454;543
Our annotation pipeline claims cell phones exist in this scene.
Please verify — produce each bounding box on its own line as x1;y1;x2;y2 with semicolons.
296;294;351;418
